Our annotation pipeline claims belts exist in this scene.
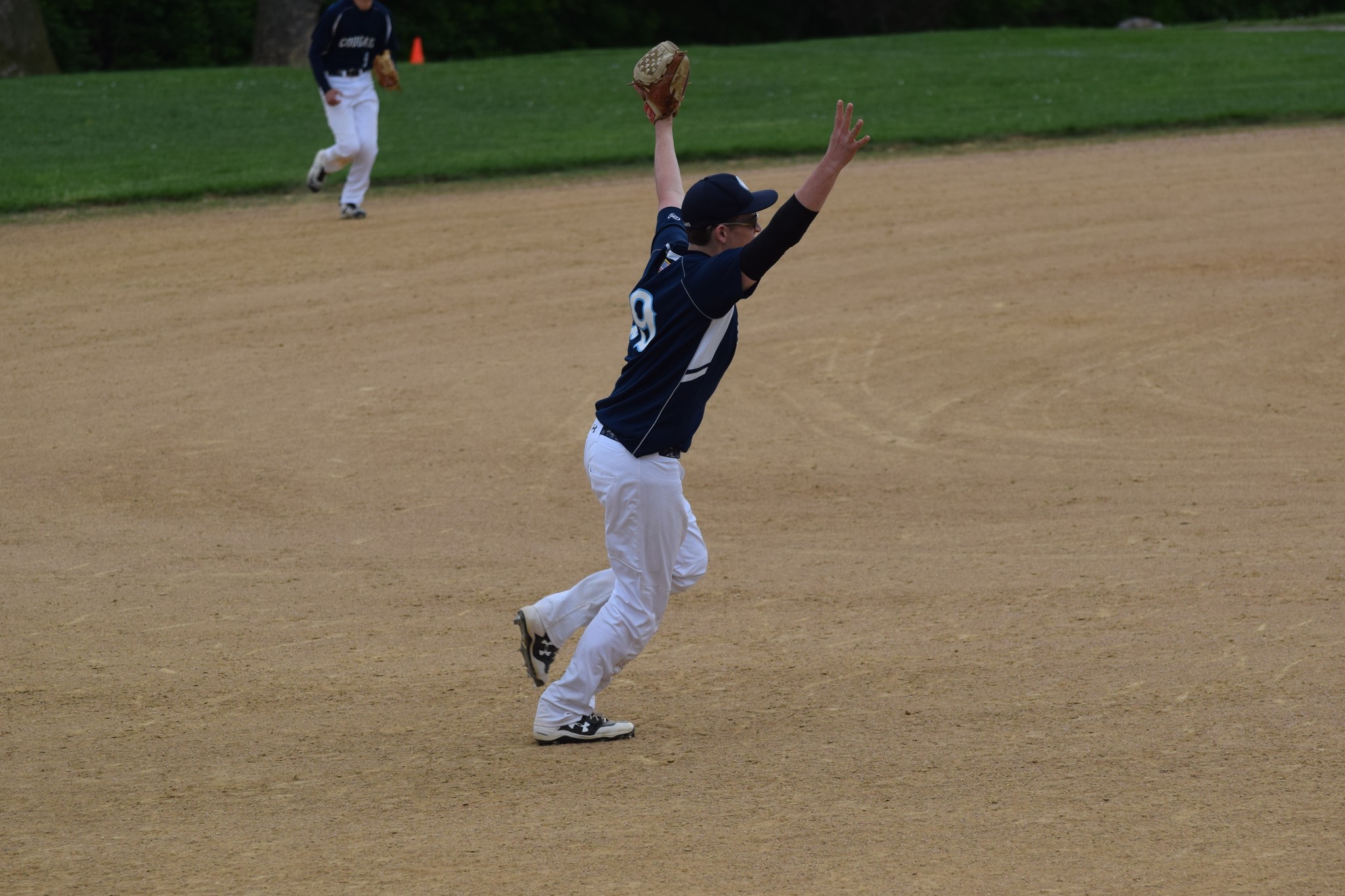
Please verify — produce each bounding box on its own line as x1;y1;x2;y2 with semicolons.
329;67;369;77
600;428;680;459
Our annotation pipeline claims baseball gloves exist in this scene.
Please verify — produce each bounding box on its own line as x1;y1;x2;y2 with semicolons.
631;40;690;125
375;50;400;91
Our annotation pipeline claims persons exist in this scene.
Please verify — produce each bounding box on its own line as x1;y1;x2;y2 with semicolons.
306;0;400;219
513;40;870;743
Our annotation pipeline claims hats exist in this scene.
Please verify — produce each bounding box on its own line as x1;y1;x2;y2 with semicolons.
681;174;779;226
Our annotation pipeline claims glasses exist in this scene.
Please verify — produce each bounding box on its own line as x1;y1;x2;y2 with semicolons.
706;214;760;229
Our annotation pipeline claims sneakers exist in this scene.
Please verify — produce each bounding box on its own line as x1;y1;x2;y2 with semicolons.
307;149;331;193
512;603;559;687
532;709;635;746
339;202;365;219
